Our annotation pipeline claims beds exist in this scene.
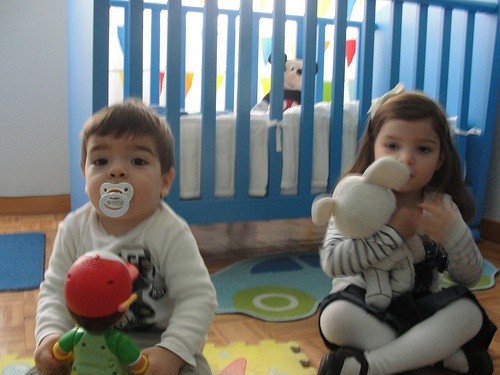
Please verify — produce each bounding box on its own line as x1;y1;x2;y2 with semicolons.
87;0;500;249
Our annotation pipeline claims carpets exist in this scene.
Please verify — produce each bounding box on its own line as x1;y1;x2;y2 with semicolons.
197;249;498;324
0;230;46;290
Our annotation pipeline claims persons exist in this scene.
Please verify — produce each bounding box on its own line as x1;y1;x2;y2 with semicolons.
316;90;498;375
26;101;218;374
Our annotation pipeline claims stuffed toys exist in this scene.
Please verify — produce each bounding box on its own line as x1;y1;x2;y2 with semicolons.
311;157;427;310
248;52;319;115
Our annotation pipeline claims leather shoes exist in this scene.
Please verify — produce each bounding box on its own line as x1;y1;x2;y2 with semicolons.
431;347;494;375
318;347;368;375
